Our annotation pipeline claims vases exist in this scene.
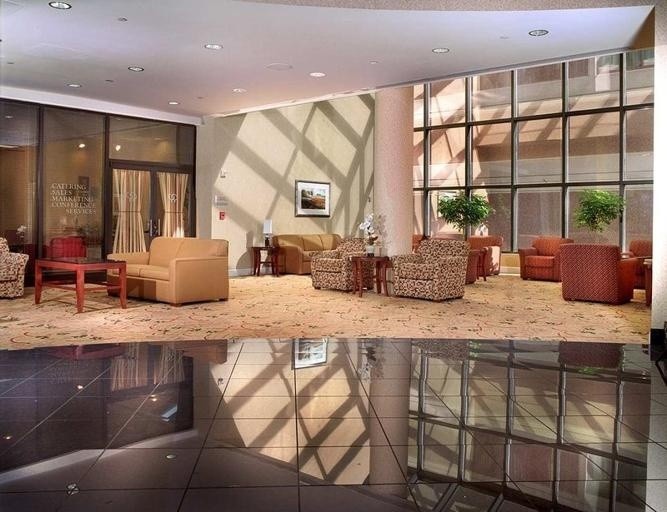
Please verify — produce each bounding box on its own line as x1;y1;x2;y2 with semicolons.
364;236;377;257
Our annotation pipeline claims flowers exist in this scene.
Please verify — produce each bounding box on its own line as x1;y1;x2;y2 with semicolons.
356;358;372;381
359;215;375;239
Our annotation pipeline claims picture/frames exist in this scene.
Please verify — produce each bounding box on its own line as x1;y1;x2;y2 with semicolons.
76;175;90;208
294;179;332;218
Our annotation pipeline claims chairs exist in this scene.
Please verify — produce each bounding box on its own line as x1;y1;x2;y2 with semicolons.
412;338;468;363
517;236;575;283
621;240;652;287
3;228;24;247
310;237;374;292
392;240;469;302
289;337;327;371
42;236;87;276
0;238;29;298
557;242;638;303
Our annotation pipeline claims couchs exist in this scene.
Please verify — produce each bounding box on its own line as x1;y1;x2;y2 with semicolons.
149;341;228;364
105;235;228;306
270;234;345;275
412;233;503;277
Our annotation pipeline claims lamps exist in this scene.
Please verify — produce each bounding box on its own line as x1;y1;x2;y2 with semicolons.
261;219;272;246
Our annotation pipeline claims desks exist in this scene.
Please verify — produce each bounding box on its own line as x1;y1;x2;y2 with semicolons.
468;247;488;281
350;255;389;298
251;246;280;278
643;259;651;306
